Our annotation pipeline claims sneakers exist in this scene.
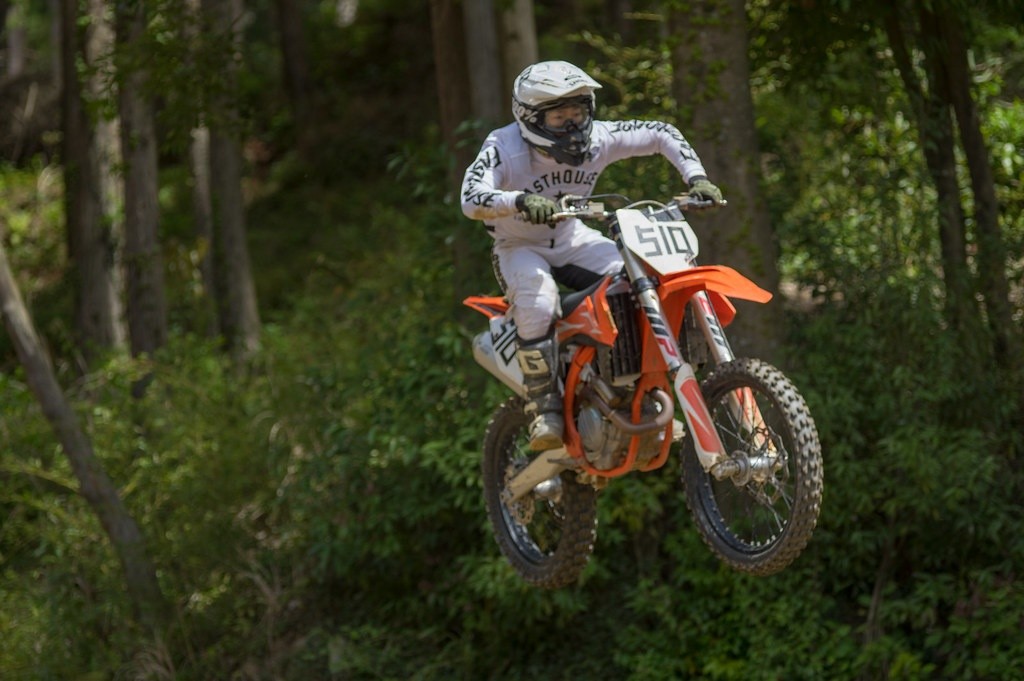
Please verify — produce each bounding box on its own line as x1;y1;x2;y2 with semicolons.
529;412;563;450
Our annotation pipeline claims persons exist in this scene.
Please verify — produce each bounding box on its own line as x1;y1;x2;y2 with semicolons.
462;61;724;452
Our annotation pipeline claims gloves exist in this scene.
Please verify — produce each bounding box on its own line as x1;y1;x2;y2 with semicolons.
515;187;566;229
688;179;723;211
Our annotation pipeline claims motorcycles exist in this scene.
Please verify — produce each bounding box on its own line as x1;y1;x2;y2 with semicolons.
463;186;824;592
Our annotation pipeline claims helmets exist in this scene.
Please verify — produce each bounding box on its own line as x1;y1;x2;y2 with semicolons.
513;60;603;167
